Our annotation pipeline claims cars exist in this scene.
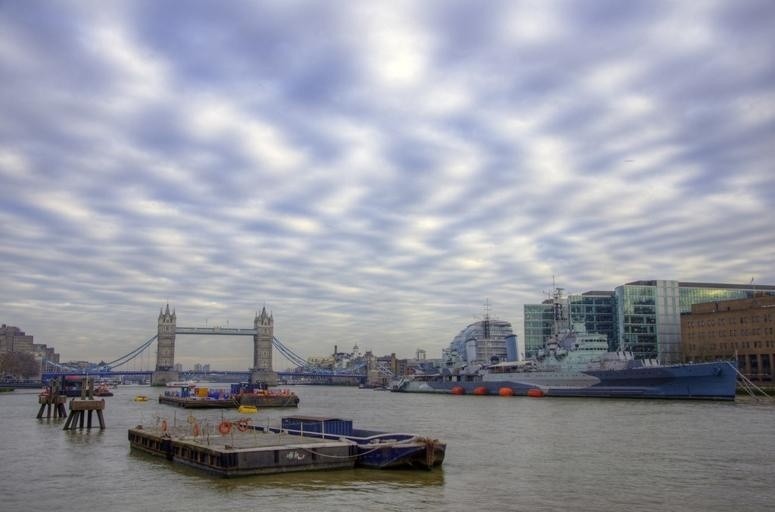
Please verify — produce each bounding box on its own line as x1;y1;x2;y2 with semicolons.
3;375;19;382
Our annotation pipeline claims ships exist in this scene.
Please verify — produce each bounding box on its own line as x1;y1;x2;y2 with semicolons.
384;285;740;402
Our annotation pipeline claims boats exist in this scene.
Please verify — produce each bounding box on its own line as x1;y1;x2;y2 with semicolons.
230;379;300;408
166;380;197;388
54;374;115;397
133;395;149;401
238;405;258;414
234;414;447;470
158;386;233;410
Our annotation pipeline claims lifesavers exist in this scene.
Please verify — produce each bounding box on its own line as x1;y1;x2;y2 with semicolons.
158;416;171;432
135;394;148;401
219;421;230;434
194;424;199;436
238;420;248;431
187;415;194;423
